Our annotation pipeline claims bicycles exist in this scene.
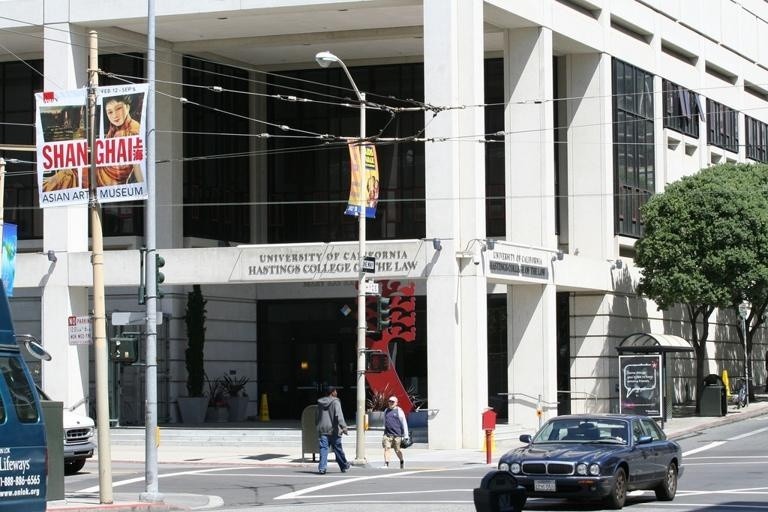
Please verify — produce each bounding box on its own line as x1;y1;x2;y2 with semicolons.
736;377;752;409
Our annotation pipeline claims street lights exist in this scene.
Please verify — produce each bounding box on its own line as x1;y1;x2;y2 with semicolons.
316;51;366;467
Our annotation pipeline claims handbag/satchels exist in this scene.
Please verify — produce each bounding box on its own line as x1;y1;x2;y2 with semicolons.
400;431;413;448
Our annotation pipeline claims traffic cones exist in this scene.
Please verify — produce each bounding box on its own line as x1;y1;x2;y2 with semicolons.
722;371;732;400
259;393;270;423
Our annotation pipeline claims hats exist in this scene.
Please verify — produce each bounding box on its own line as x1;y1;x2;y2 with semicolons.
323;386;336;394
389;396;398;403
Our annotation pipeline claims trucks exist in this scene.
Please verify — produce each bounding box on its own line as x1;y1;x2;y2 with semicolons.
0;282;50;512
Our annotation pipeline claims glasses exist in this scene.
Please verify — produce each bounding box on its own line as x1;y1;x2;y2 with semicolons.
388;400;395;403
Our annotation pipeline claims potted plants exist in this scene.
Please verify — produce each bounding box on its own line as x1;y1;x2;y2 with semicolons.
176;285;250;424
365;392;387;427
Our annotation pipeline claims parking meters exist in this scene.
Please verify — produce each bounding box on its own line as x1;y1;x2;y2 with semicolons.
474;469;526;512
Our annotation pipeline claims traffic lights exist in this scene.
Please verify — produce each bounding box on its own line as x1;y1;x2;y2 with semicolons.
156;253;165;300
377;295;392;330
371;353;390;371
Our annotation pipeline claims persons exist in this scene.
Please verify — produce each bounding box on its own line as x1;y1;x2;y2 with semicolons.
382;395;411;469
42;92;143;192
313;386;351;474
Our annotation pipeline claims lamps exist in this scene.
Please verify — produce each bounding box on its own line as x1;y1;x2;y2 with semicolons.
432;237;442;251
47;249;58;263
555;250;564;261
482;239;494;251
615;259;622;269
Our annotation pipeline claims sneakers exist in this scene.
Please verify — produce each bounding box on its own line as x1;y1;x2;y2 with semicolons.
341;462;352;473
319;469;327;475
382;461;404;469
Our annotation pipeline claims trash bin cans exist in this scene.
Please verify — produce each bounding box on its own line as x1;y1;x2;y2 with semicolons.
699;373;727;417
301;404;338;462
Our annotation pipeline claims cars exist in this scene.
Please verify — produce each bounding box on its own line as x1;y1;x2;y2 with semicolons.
35;385;93;473
499;413;681;509
